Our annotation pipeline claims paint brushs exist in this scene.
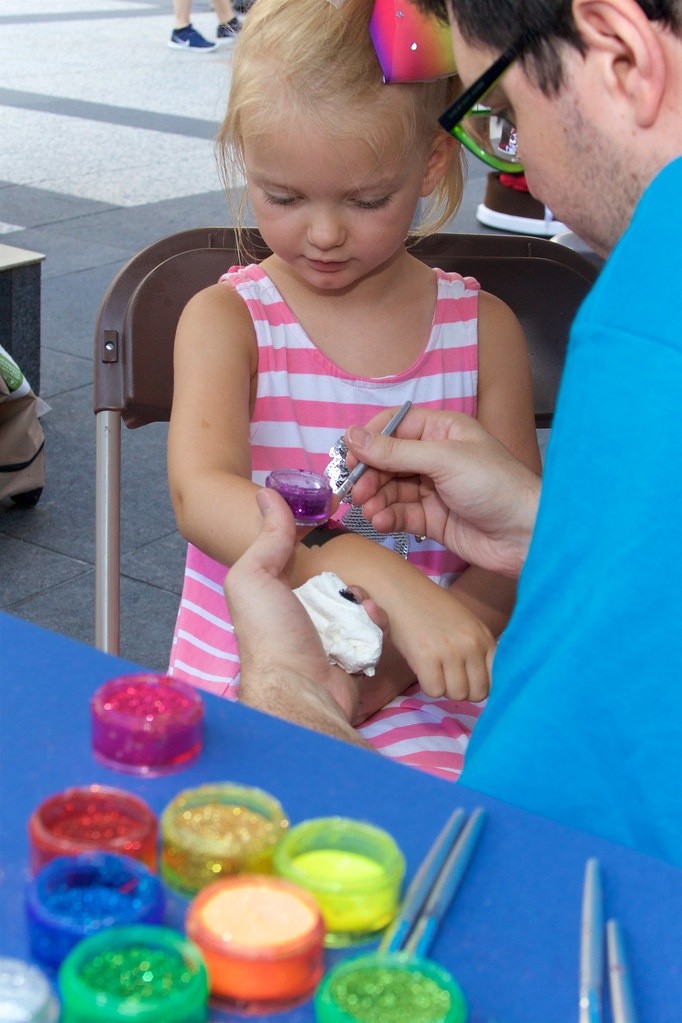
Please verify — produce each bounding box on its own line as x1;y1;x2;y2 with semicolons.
577;857;637;1023
370;805;488;954
331;400;412;514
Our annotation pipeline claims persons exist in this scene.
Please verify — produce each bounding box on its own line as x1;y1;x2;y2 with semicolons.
166;0;543;781
223;0;682;867
167;0;244;54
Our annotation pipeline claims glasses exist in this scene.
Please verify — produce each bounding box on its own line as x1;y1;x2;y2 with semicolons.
438;37;537;174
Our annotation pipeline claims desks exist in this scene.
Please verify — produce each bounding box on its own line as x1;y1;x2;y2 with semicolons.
0;613;682;1023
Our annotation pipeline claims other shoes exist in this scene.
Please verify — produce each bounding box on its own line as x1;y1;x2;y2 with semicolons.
168;24;219;52
217;17;243;43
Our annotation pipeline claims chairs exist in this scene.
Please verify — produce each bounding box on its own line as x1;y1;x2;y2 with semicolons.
93;228;602;656
